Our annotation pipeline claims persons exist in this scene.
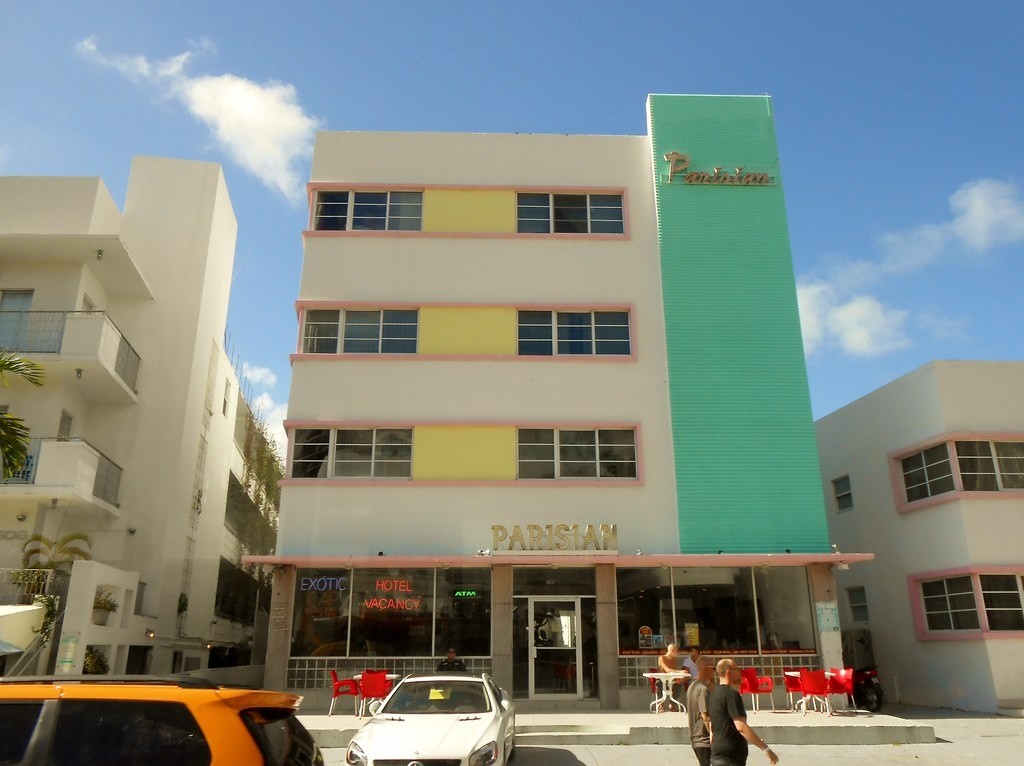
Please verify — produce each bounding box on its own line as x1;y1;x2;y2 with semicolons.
654;643;688;712
682;644;715;692
686;656;716;766
708;659;779;766
436;647;467;671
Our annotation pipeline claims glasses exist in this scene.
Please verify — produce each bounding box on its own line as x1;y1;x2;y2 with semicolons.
730;668;741;672
706;666;716;670
449;651;455;653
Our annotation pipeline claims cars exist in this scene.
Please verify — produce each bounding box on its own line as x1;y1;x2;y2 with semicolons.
344;673;516;766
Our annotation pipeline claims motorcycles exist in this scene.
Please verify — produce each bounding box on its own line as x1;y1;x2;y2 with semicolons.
850;664;883;713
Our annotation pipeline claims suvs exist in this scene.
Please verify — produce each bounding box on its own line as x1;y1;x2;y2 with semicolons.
0;676;324;766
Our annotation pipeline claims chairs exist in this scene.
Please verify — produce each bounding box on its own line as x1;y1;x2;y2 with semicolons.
553;664;577;693
365;668;390;709
454;697;479;710
741;668;775;714
359;671;393;719
799;668;832;717
649;667;680;713
328;669;360;716
828;668;857;715
782;667;818;712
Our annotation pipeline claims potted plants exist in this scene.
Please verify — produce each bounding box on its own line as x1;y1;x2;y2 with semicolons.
14;565;70;605
92;589;119;627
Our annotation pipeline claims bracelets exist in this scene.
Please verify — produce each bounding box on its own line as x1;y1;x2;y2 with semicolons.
762;746;769;753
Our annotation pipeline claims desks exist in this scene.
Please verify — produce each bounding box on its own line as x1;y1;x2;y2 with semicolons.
353;674;400;716
784;672;836;712
643;673;691;714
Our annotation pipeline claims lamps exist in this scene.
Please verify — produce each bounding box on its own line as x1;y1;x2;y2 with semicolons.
555;610;560;617
837;562;850;571
277;564;286;577
203;642;211;649
97;250;103;261
831;543;840;554
476;549;490;557
76;368;82;380
51;498;57;510
146;629;154;639
547;608;553;616
544;576;555;584
